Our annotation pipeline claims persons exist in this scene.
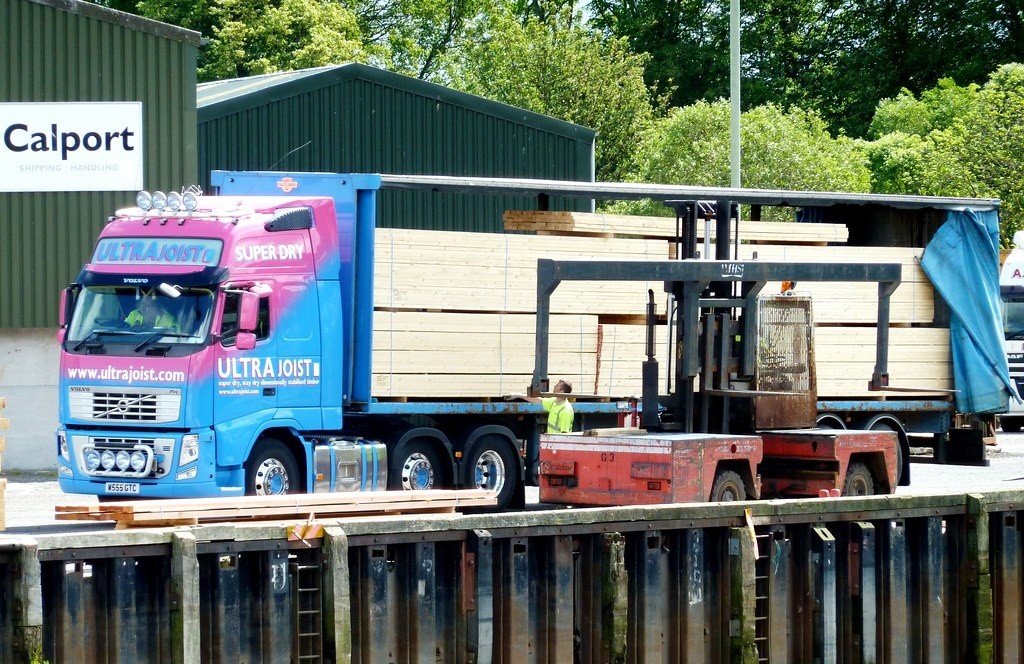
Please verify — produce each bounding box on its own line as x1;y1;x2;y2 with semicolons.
124;295;181;334
504;379;574;434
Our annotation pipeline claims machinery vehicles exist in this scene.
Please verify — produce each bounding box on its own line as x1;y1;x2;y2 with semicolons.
524;199;904;506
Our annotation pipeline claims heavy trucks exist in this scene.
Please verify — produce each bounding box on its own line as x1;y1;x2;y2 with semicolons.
56;168;1022;499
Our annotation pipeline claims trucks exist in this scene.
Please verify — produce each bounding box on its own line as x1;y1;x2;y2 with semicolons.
996;228;1024;434
